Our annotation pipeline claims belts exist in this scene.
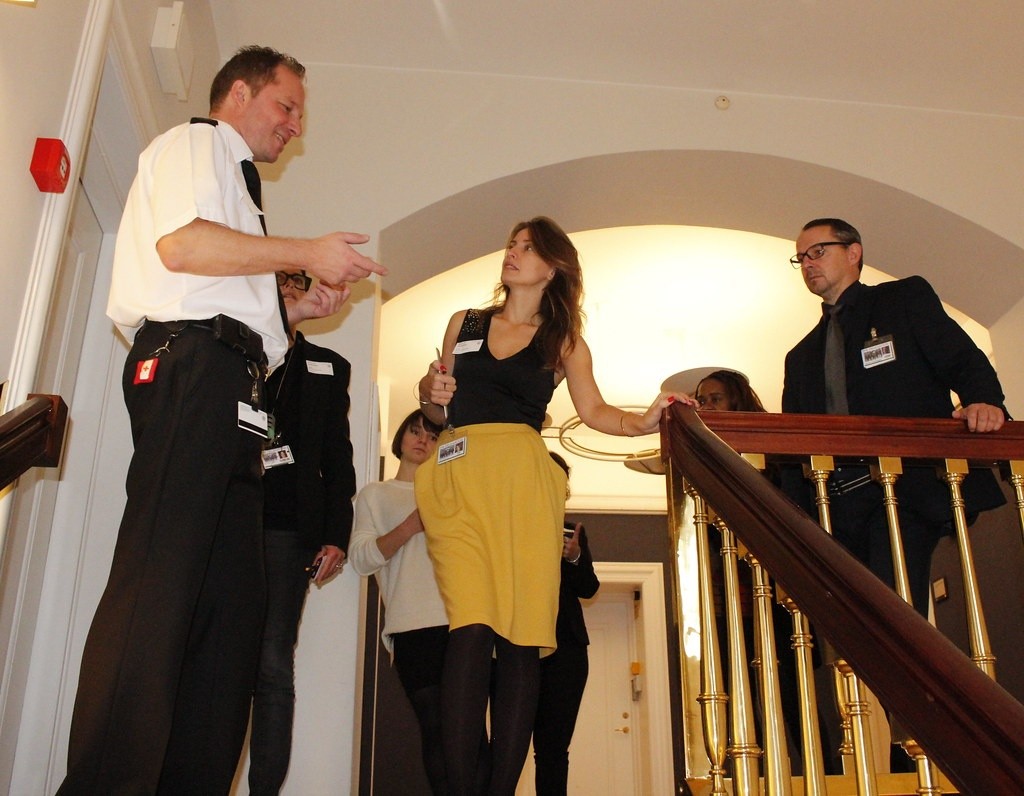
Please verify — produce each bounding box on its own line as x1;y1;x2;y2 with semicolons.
188;318;214;332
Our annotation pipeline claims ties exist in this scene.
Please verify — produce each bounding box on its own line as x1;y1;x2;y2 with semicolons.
824;304;849;416
240;160;295;343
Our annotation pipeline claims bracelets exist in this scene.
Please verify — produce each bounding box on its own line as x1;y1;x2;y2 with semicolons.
570;547;581;562
413;381;431;404
621;411;633;437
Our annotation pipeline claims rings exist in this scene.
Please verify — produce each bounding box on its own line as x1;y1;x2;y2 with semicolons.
336;564;343;570
444;383;446;391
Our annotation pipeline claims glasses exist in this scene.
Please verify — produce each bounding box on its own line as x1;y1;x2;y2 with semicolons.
275;270;313;292
789;242;851;269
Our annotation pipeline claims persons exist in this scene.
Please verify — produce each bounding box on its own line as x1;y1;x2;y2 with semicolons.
695;370;844;775
55;46;391;796
782;218;1006;775
455;443;463;452
280;451;286;458
350;216;699;796
883;348;890;354
